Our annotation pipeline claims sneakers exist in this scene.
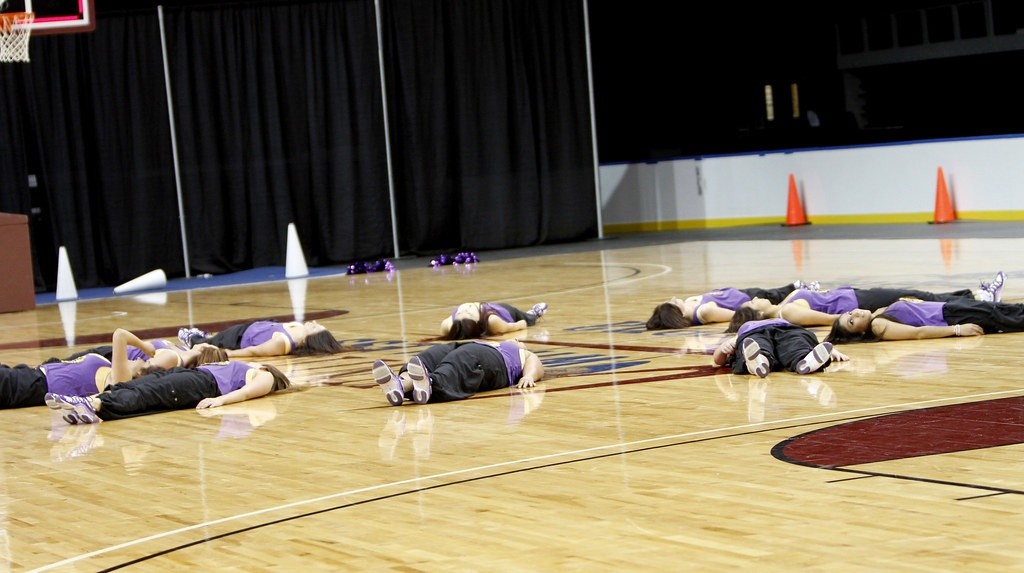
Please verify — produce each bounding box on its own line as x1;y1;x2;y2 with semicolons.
44;392;97;413
189;328;209;338
527;303;548;319
976;278;991;294
63;407;103;424
372;359;406;406
807;280;821;290
407;356;433;404
793;279;804;289
985;271;1007;303
178;327;196;349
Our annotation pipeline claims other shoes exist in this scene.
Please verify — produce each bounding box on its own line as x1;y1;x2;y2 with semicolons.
742;338;770;378
796;342;833;374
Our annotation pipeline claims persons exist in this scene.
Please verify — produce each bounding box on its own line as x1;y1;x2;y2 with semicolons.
372;339;547;407
713;316;851;380
724;269;1006;334
823;299;1024;349
176;318;350;358
0;326;184;410
645;278;821;331
40;334;230;369
44;360;292;426
440;300;549;339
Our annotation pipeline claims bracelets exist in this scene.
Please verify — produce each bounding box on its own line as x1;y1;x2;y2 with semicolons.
954;324;960;337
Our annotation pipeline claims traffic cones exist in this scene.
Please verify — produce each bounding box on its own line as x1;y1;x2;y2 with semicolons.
58;301;77;346
780;173;811;226
286;223;309;278
114;269;168;294
928;167;959;225
56;245;79;300
288;278;307;323
